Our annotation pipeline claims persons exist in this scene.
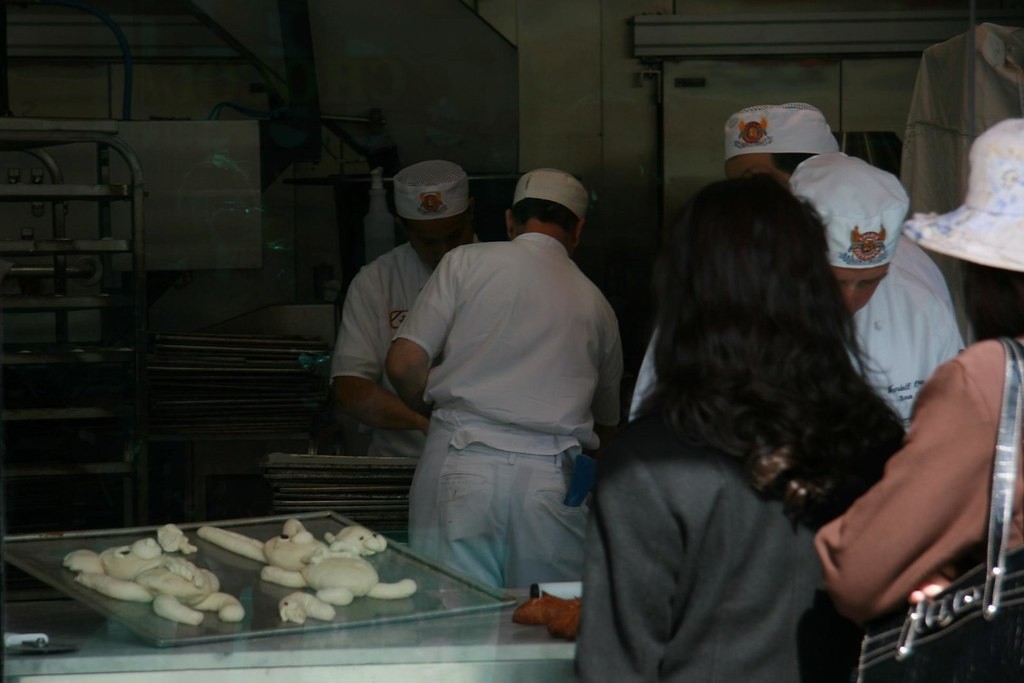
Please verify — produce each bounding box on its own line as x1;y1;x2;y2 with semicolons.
812;119;1024;683
329;158;478;458
575;176;904;683
627;155;962;432
384;166;625;594
718;100;841;189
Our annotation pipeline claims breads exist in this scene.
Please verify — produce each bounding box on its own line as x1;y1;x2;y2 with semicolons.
513;594;582;639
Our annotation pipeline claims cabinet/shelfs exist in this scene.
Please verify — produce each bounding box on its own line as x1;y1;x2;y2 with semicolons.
0;130;147;603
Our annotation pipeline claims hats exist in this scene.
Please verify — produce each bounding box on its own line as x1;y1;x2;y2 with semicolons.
512;168;588;219
394;160;469;220
902;117;1024;272
722;102;839;163
788;151;910;268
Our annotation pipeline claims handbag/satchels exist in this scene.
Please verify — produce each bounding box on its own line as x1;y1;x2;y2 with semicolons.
855;337;1024;683
